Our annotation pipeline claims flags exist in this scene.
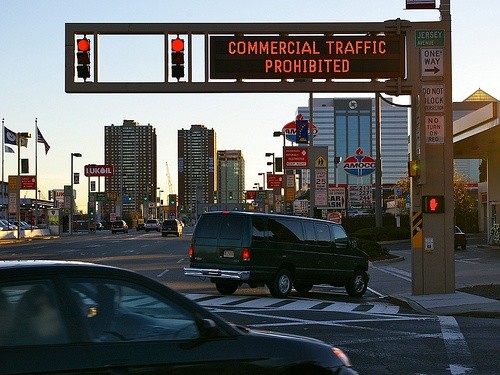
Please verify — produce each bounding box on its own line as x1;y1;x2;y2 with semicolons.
38;127;50;155
5;146;15;153
4;127;17;145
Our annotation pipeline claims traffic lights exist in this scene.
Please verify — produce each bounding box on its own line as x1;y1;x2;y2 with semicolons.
90;212;94;221
214;195;217;200
229;192;233;199
76;37;92;78
425;195;442;211
172;39;185;77
128;197;132;200
144;197;147;201
170;200;174;204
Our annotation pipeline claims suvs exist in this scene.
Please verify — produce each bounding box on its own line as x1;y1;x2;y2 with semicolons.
144;219;160;232
112;220;128;233
183;210;369;299
161;219;183;237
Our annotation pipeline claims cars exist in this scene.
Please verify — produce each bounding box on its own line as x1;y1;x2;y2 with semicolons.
457;226;467;249
0;259;358;375
0;219;38;231
136;223;146;231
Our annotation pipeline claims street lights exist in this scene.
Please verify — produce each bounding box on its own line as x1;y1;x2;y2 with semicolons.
17;132;32;239
70;152;82;235
252;153;276;210
273;130;288;214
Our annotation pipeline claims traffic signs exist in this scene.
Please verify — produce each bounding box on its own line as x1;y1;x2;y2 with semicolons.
421;48;444;77
